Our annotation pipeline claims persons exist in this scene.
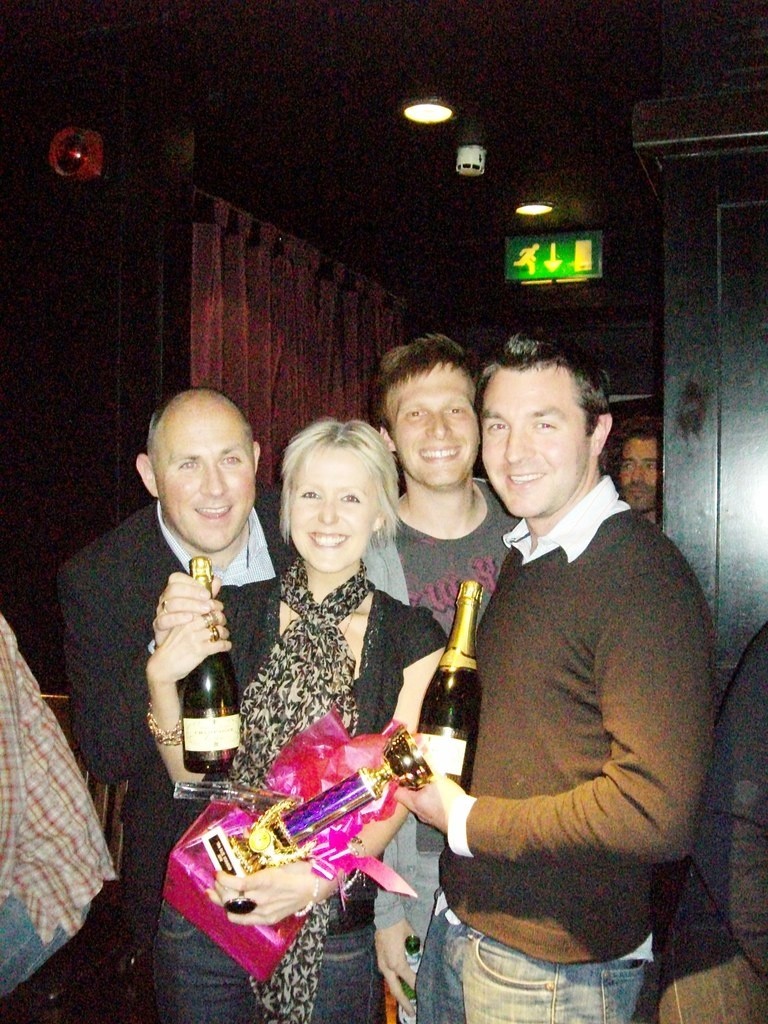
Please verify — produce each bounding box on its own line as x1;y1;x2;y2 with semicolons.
618;415;656;526
395;327;709;1023
656;622;767;1024
0;614;116;1001
61;386;298;972
144;417;449;1024
361;334;525;1021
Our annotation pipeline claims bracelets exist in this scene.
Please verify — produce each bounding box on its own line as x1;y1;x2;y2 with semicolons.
333;836;366;899
294;875;319;917
147;701;183;746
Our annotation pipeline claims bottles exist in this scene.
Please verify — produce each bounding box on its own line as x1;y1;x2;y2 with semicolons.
181;555;242;772
415;581;482;798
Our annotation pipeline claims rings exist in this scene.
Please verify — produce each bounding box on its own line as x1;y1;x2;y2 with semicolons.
161;601;168;615
209;625;219;642
224;891;256;914
202;611;218;628
